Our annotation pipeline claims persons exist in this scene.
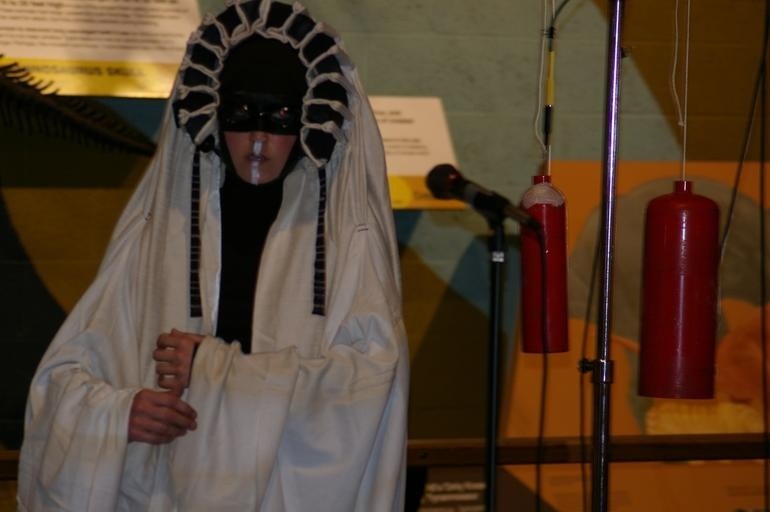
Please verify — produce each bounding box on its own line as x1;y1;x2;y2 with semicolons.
15;2;413;512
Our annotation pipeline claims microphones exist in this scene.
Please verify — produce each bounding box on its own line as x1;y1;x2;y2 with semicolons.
424;164;542;231
